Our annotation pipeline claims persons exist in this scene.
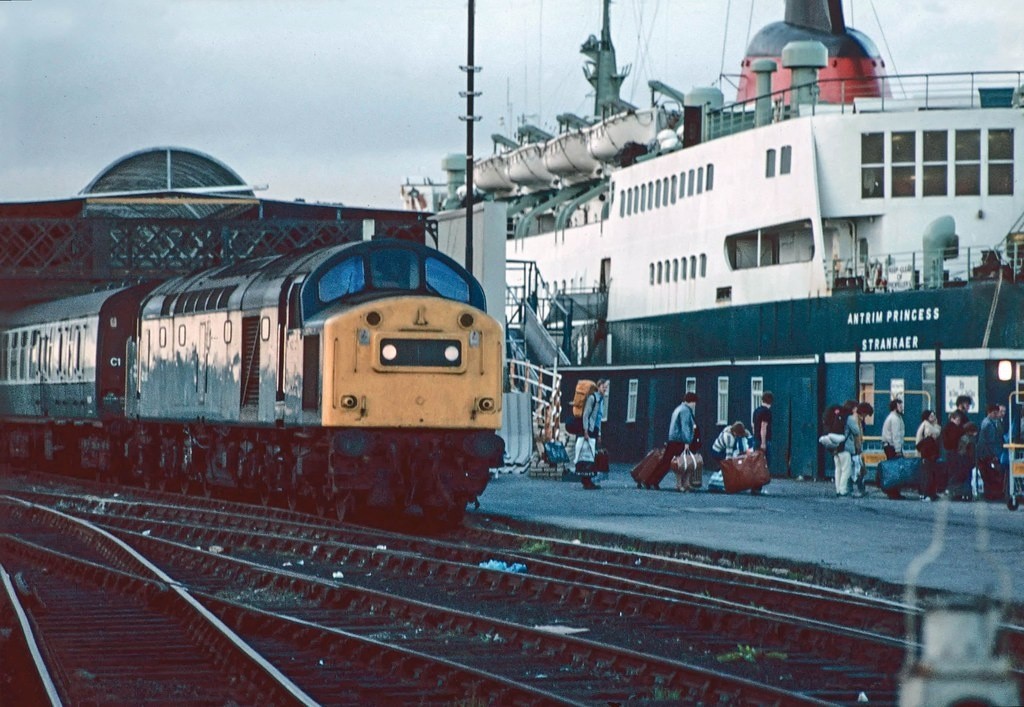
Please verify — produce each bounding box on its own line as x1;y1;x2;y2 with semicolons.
914;409;942;499
577;378;608;491
736;429;754;453
829;400;873;498
942;395;1006;502
752;393;773;495
711;422;745;459
882;399;906;499
668;392;698;492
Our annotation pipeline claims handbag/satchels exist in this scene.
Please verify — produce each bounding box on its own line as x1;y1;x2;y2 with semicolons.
876;453;931;493
691;426;703;450
720;449;771;494
595;445;610;473
575;459;597;477
671;447;704;491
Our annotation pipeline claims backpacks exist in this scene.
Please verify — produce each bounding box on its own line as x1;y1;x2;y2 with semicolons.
822;403;855;454
565;377;599;435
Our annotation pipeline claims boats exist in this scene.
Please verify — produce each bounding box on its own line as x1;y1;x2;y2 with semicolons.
582;104;684;165
537;128;605;183
470;154;518;193
504;142;561;190
413;0;1023;492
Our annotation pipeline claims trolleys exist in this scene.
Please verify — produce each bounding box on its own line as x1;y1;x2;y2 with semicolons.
1008;391;1024;509
858;389;931;468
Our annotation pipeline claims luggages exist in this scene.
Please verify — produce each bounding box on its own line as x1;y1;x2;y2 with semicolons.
630;446;673;490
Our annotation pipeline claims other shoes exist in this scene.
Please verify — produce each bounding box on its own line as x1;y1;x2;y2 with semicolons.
581;475;601;490
836;493;868;499
751;489;769;496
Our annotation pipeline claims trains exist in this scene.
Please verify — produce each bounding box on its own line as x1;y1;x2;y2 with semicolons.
0;237;505;529
